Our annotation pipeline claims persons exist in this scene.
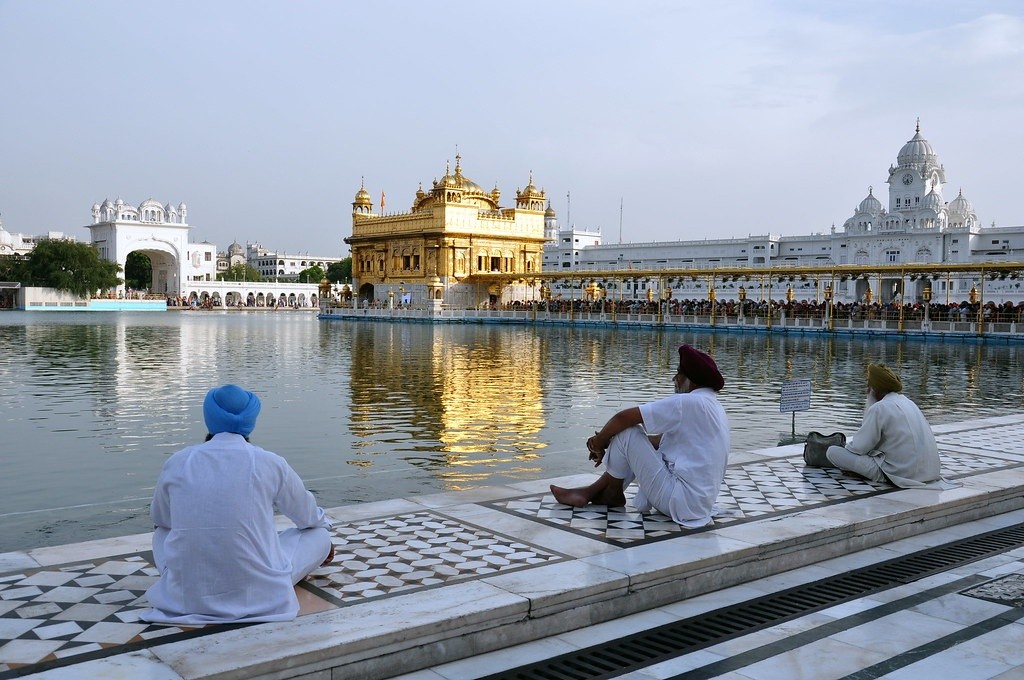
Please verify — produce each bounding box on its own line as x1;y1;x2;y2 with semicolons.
481;297;1023;324
96;286;134;298
140;385;334;628
165;294;308;307
825;363;941;485
333;297;409;309
550;344;730;528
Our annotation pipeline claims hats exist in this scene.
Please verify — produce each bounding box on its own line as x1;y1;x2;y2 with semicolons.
866;362;903;393
203;384;261;435
677;344;724;391
